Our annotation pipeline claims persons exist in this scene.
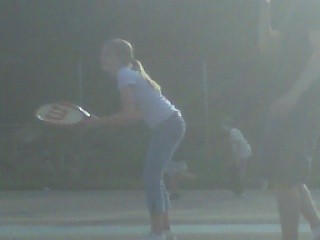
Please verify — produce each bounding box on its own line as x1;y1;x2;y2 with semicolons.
78;39;187;239
265;51;320;240
222;118;251;200
